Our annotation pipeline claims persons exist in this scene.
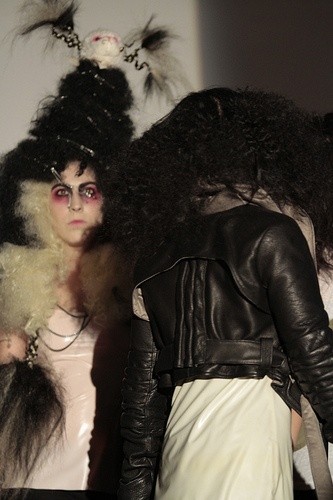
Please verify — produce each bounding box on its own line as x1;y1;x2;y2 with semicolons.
0;0;333;500
124;89;333;500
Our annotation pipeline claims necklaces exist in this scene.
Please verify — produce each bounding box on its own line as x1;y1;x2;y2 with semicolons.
34;303;92;352
